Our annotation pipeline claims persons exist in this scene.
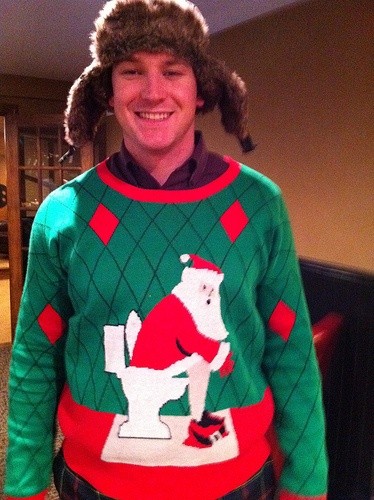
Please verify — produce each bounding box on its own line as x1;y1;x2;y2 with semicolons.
5;0;331;500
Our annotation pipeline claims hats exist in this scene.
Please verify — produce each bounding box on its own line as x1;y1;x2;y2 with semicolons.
63;0;248;149
